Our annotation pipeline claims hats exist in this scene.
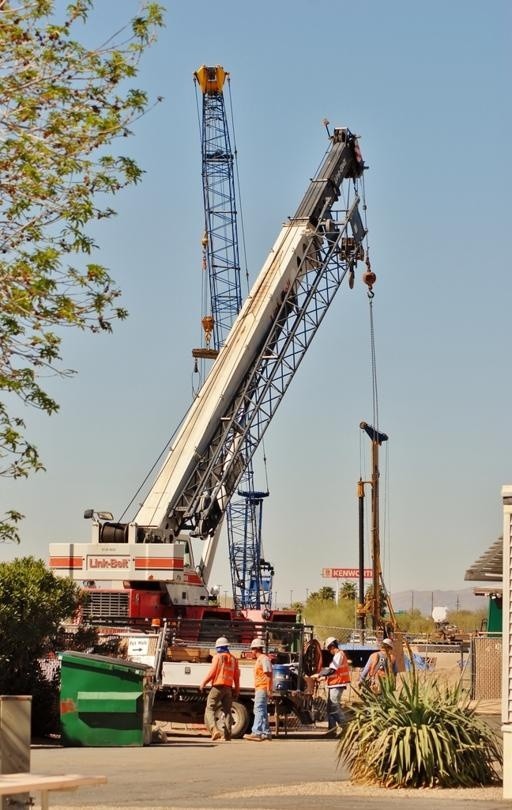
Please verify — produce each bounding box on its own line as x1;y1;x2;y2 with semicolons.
215;637;231;647
251;638;265;648
383;638;394;649
325;636;336;649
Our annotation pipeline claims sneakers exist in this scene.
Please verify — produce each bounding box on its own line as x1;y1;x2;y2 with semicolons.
243;733;272;741
210;732;231;741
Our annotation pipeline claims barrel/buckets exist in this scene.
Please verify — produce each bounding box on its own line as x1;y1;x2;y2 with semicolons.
271;664;290;689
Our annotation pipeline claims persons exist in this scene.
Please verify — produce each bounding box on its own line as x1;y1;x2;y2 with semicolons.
309;636;351;738
242;638;274;743
356;638;398;696
199;636;241;741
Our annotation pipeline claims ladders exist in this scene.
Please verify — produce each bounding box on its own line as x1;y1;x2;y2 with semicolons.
452;649;470;699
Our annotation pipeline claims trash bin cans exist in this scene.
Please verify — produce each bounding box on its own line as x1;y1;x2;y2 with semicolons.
60;650;153;747
